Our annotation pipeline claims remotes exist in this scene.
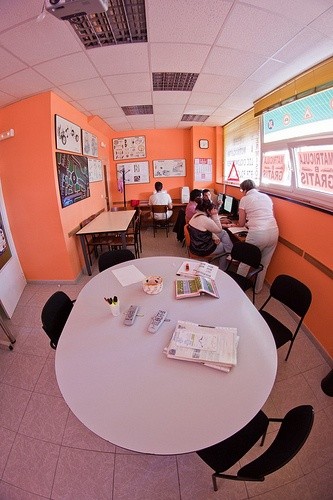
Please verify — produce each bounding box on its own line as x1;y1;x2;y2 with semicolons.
124;305;138;326
147;310;167;333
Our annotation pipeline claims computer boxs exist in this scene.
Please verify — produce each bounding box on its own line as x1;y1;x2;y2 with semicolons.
181;187;190;204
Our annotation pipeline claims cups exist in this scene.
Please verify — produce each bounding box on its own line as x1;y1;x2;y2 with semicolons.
109;302;120;316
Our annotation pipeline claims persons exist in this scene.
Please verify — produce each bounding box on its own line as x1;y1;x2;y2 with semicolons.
237;179;279;294
149;182;173;220
186;198;239;272
184;188;224;224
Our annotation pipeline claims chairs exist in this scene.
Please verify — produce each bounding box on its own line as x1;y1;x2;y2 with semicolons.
41;205;315;492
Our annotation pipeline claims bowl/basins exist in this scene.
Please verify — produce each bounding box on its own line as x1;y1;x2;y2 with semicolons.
142;276;163;294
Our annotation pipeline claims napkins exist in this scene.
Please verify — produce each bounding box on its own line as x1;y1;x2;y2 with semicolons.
112;265;146;287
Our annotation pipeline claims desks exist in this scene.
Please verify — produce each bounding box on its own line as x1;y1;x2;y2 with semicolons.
55;252;278;456
138;203;187;227
75;210;136;276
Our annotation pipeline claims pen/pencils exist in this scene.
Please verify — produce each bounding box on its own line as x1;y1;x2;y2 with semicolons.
104;297;108;302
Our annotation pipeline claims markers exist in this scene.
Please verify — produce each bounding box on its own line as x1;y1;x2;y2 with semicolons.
108;296;118;305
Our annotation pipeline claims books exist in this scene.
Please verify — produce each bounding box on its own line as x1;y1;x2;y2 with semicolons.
164;259;240;373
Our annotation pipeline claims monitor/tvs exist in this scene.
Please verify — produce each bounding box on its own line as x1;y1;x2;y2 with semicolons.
153;190;167;194
218;193;240;220
